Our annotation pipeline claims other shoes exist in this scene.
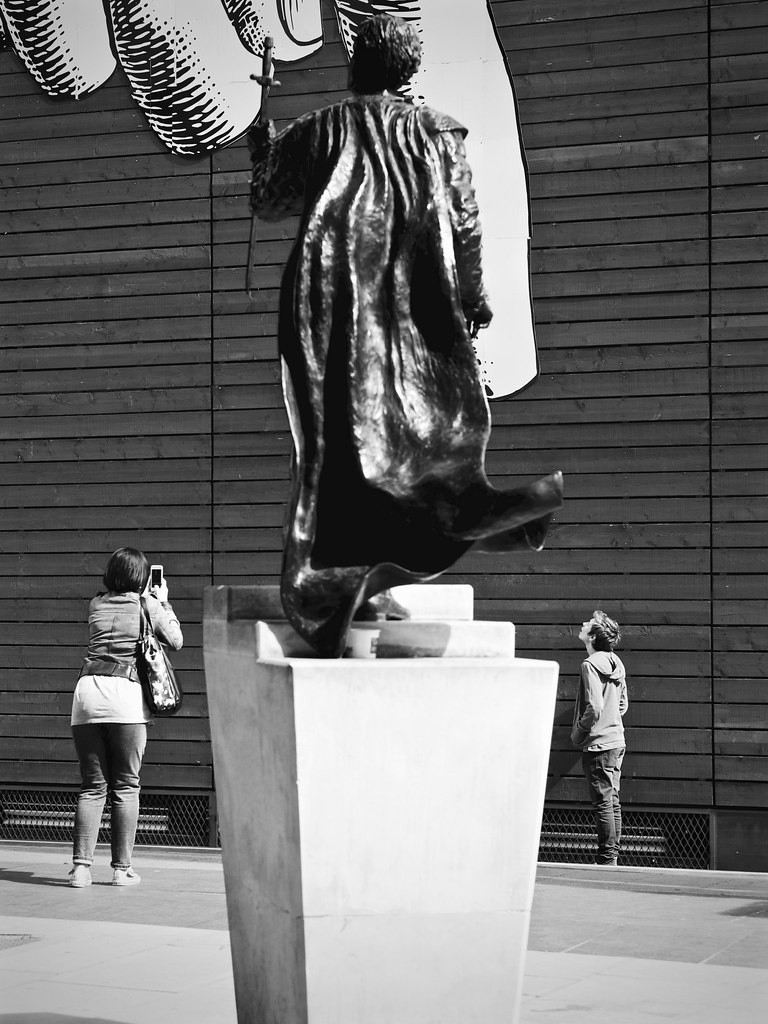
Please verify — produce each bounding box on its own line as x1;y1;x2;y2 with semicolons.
595;856;618;865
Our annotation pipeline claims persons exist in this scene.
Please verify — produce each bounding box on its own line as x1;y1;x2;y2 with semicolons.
243;17;566;658
66;548;184;887
571;610;628;865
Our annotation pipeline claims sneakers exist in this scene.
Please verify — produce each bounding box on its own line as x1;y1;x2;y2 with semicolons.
67;865;93;888
112;866;141;886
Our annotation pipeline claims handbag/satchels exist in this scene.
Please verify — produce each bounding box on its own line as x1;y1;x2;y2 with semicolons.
136;596;183;717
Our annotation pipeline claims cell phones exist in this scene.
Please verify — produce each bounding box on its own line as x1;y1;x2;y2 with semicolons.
151;564;163;591
